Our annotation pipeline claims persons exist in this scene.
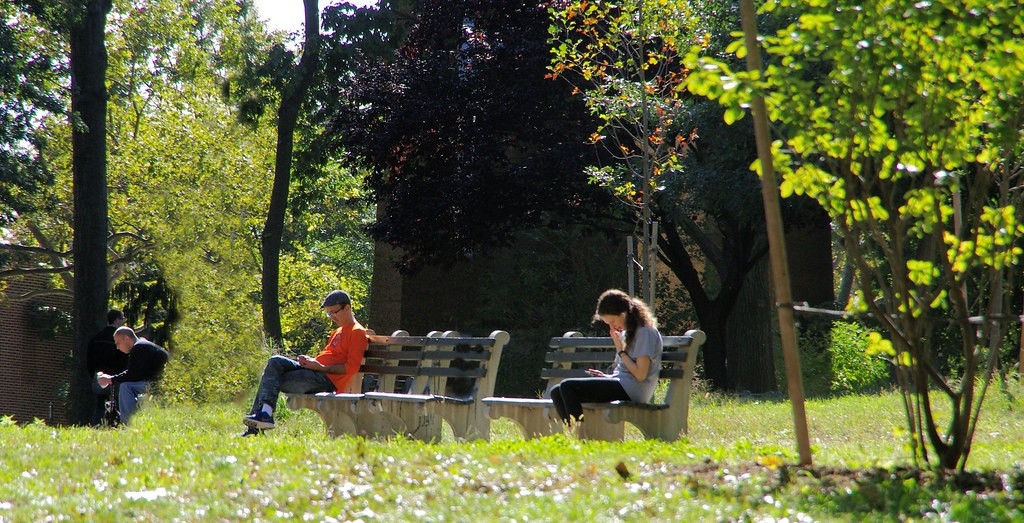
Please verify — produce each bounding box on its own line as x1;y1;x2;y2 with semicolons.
80;309;169;425
240;289;367;437
550;289;664;438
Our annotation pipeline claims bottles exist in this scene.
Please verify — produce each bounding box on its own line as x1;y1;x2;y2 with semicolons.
97;371;109;388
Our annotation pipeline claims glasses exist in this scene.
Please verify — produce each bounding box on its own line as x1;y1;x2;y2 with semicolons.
326;309;341;317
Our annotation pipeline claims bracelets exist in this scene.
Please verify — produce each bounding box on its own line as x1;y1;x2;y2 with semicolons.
617;350;626;358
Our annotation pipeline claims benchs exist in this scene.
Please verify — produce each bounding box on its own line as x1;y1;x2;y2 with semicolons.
286;330;509;446
481;330;705;444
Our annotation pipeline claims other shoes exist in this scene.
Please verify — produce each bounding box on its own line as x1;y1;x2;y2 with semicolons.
241;431;264;437
243;411;274;429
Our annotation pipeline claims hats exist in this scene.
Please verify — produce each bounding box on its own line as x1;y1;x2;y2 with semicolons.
320;290;350;308
